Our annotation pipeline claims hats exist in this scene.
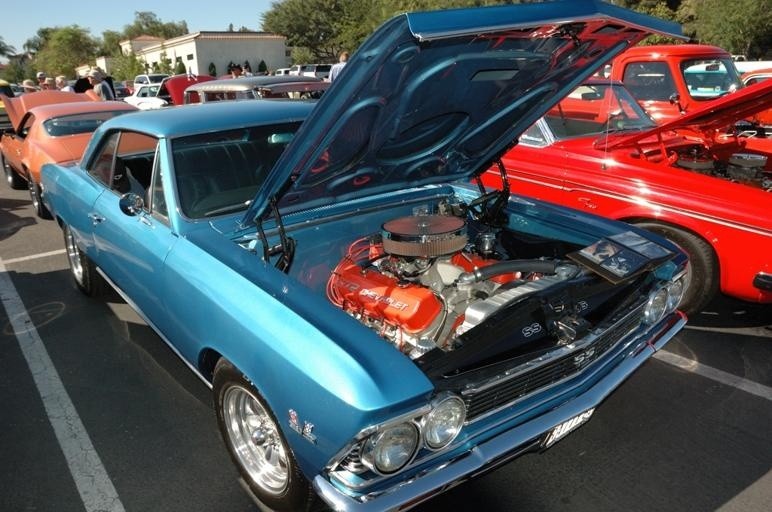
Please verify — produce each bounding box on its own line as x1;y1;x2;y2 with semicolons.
37;72;45;78
79;69;90;77
42;78;53;85
17;80;36;90
88;70;101;82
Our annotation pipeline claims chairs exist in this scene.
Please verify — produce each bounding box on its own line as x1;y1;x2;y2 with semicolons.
117;139;271;206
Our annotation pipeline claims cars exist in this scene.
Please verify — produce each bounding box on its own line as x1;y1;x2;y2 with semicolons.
41;1;694;511
3;1;770;329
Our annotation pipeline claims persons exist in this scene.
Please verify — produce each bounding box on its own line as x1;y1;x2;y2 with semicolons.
22;63;117;103
328;51;349;84
241;65;252;75
228;63;242;80
